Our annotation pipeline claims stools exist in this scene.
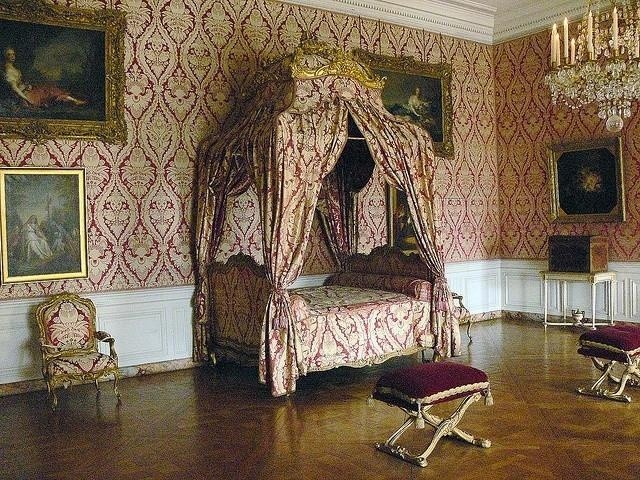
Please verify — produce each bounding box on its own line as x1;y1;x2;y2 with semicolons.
577;319;640;403
368;361;493;468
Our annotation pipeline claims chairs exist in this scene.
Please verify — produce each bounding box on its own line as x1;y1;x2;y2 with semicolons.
35;290;122;411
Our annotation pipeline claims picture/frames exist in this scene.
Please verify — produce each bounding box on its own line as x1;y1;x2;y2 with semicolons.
1;0;130;147
0;165;90;286
543;136;626;225
352;48;457;160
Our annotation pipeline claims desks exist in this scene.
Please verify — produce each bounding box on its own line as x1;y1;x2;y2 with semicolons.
537;270;620;329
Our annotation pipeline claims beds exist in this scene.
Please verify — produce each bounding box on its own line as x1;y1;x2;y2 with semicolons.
204;236;442;400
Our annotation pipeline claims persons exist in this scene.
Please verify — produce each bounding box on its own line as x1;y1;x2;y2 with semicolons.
409;87;433;131
2;46;89;108
18;215;53;262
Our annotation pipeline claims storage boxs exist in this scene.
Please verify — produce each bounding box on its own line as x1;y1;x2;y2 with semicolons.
547;234;609;275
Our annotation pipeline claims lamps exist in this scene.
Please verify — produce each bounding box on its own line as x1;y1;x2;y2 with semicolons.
543;0;639;130
